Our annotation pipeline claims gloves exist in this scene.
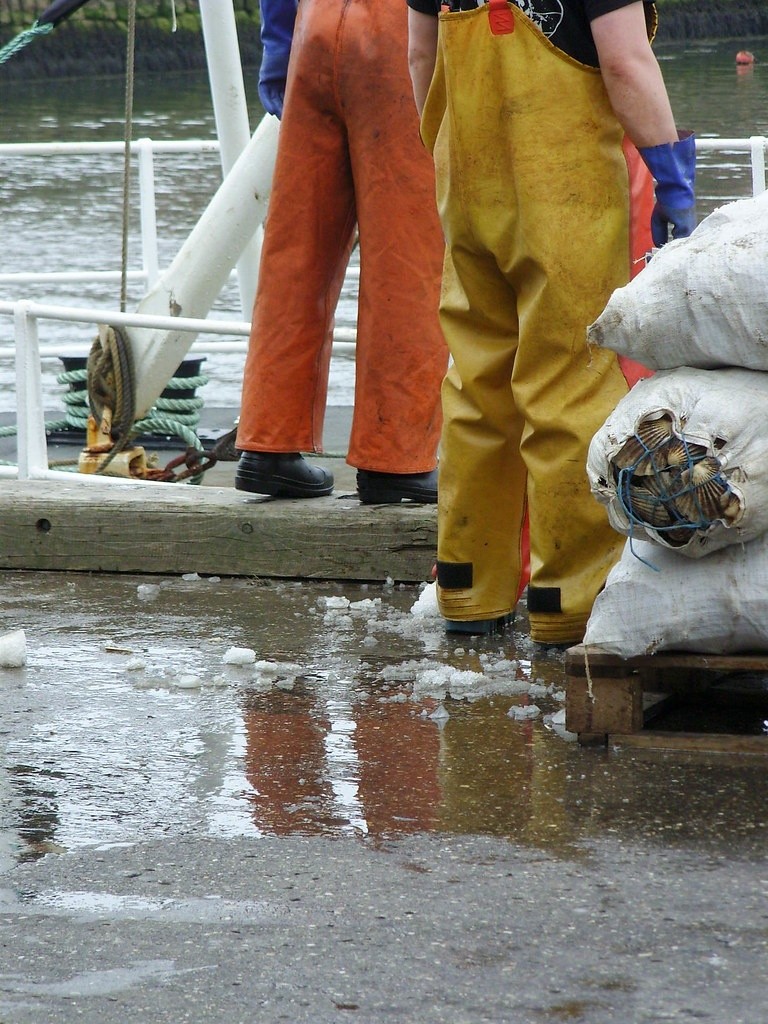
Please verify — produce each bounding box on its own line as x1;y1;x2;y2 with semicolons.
258;0;300;121
635;131;697;263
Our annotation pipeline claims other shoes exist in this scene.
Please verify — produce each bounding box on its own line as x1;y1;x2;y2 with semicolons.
356;466;440;502
235;451;335;497
445;612;515;633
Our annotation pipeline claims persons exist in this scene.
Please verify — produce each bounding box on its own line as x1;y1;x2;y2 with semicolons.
402;0;695;653
211;0;664;612
235;669;570;849
